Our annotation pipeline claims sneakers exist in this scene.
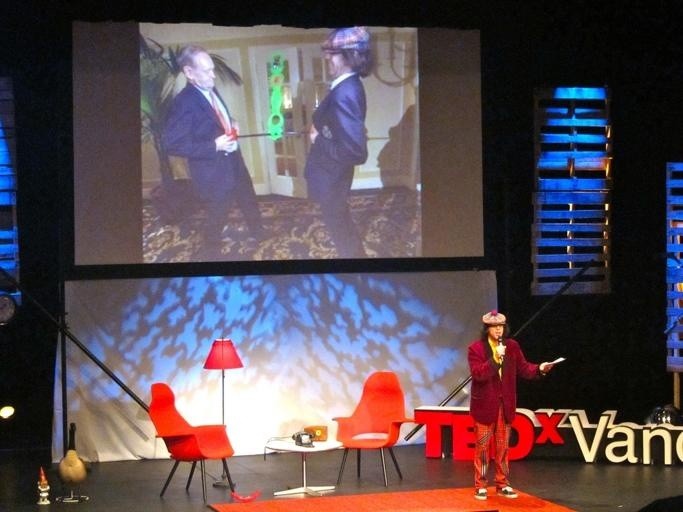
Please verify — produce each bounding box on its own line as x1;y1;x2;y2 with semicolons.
474;486;518;500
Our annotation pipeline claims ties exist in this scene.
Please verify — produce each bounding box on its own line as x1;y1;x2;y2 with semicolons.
209;90;231;136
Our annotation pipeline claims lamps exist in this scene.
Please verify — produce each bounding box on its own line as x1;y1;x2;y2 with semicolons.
203;334;243;487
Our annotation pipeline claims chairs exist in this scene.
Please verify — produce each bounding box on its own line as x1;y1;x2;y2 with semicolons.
330;371;419;487
147;382;236;503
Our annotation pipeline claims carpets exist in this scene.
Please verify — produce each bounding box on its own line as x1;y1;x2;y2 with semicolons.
205;482;581;511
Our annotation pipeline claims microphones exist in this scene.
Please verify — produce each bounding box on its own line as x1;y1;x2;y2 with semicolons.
498;336;505;362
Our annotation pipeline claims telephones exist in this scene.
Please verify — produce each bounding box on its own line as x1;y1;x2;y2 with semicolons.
292;431;314;446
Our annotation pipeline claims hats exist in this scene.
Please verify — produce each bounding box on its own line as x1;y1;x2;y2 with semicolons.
321;26;370;52
482;310;506;325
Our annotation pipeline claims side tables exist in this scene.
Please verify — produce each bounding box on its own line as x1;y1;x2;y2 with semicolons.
264;437;344;499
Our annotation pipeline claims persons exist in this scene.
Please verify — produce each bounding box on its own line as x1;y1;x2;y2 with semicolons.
163;45;278;262
468;310;554;500
303;25;374;259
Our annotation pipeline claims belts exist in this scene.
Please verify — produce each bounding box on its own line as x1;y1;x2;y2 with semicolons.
223;151;236;157
